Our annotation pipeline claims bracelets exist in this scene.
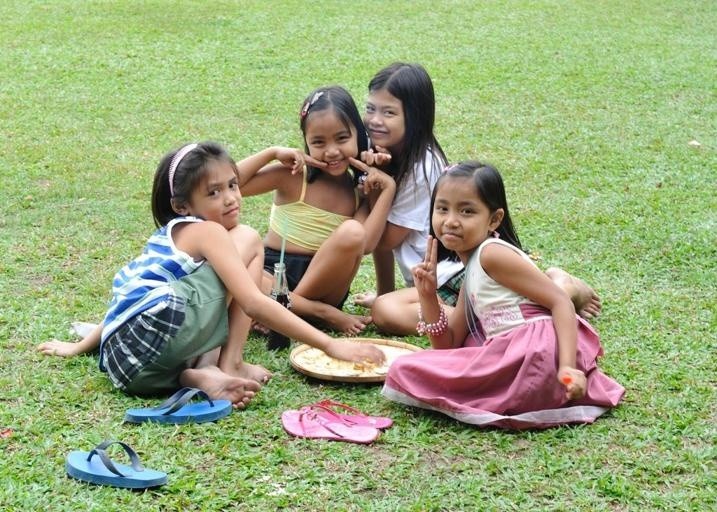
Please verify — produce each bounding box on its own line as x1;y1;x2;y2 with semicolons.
416;302;448;337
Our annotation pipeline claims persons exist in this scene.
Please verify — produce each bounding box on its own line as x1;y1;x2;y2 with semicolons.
235;84;396;337
353;60;604;335
40;139;386;410
380;158;625;431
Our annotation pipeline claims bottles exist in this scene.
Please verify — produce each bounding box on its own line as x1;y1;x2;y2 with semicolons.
268;263;292;350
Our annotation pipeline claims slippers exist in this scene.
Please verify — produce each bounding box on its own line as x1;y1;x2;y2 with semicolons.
299;400;392;429
125;387;232;424
282;406;378;444
65;441;168;488
